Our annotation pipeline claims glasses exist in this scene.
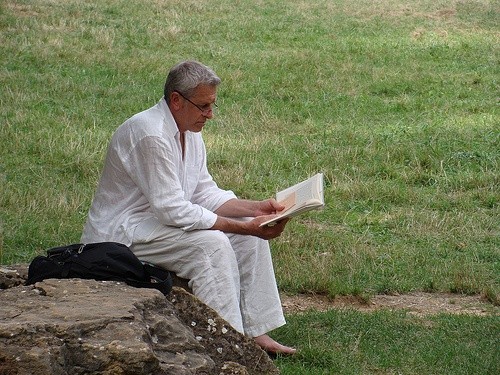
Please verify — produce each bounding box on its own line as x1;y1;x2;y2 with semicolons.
173;89;220;115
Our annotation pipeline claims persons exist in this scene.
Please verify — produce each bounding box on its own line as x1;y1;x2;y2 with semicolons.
81;60;297;354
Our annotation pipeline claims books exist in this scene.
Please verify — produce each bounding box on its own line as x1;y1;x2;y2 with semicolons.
259;172;325;228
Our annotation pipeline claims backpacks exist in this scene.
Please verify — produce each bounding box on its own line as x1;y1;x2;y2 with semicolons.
23;242;175;295
